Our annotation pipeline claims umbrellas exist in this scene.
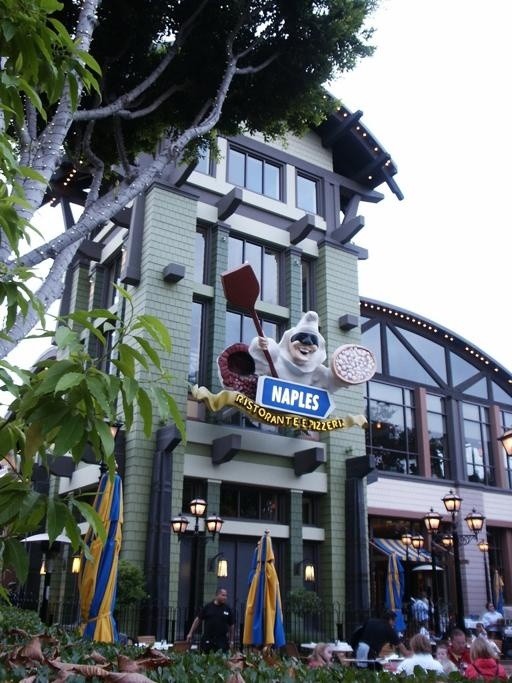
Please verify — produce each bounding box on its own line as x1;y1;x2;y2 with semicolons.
75;457;124;644
491;571;505;614
383;551;407;635
241;527;288;653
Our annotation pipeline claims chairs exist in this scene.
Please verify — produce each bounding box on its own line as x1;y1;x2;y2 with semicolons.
285;640;302;663
137;636;193;654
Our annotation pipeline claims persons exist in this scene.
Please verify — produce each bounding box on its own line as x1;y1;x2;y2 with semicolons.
434;642;448;667
442;628;471;669
464;636;508;679
481;600;507;660
394;633;445;676
347;608;408;673
475;622;487;638
185;586;237;656
411;592;434;630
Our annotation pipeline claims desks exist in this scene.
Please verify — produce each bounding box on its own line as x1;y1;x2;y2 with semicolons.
300;643;352;663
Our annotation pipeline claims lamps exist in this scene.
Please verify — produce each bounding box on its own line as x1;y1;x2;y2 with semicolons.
208;550;228;579
294;558;315;583
72;550;81;575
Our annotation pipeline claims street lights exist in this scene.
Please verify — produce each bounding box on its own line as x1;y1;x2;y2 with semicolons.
401;489;491;647
171;497;224;640
19;532;72;624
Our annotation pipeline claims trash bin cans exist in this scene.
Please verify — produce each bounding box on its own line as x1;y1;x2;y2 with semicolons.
502;625;512;657
